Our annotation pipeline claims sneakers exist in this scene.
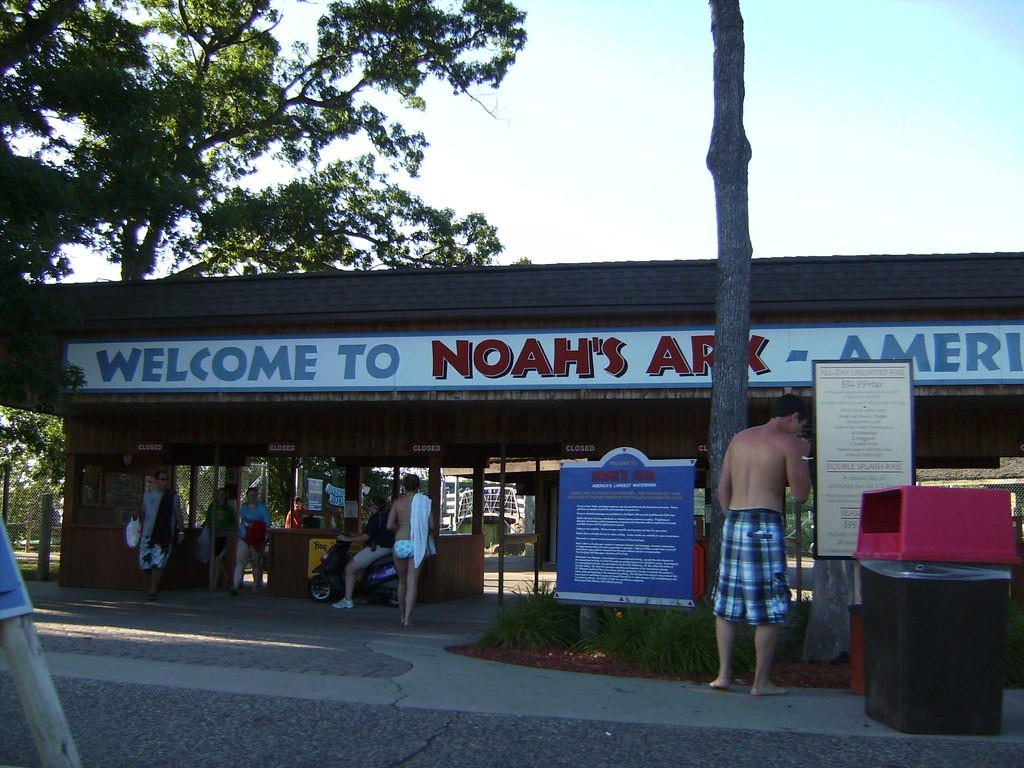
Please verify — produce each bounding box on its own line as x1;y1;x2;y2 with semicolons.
332;597;354;608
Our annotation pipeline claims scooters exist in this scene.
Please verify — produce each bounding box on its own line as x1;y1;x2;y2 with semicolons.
309;529;402;608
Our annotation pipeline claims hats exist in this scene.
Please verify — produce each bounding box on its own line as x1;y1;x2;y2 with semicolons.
371;496;386;508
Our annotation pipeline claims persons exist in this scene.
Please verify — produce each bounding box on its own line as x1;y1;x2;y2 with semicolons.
204;489;237;591
709;395;811;695
137;470;185;600
285;496;308;528
387;475;434;627
230;487;271;595
332;497;395;609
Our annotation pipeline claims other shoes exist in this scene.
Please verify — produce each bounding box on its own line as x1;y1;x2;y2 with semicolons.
252;589;259;593
227;586;239;595
147;593;159;600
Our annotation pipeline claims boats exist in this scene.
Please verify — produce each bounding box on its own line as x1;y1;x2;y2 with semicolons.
457;487;519;549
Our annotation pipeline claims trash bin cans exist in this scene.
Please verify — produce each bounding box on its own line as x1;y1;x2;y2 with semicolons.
852;485;1023;736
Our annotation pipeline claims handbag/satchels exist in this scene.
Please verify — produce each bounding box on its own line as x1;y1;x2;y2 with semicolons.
194;526;211;563
126;516;141;548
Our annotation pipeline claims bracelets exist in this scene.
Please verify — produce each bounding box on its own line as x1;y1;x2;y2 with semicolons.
179;532;184;534
802;456;814;461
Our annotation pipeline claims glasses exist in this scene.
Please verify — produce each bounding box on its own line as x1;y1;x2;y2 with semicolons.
367;504;373;508
161;478;169;481
